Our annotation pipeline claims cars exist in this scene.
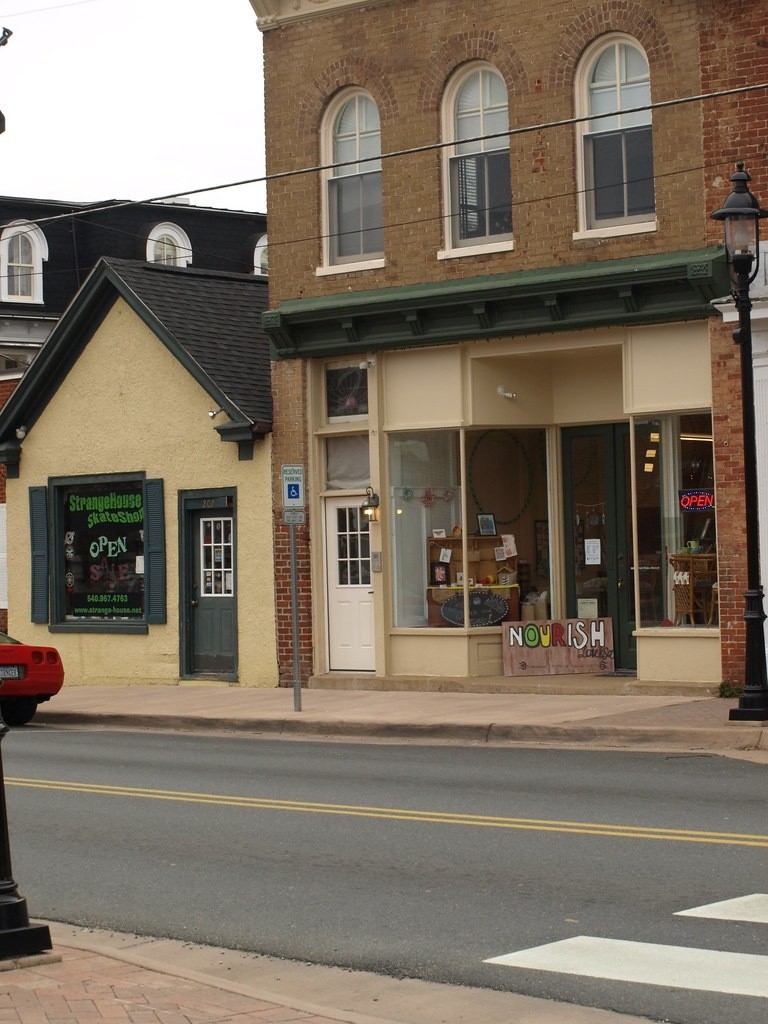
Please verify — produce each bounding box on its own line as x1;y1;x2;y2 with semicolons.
0;632;64;725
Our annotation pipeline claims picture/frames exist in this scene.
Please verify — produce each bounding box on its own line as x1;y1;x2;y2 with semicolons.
476;513;497;536
430;561;450;587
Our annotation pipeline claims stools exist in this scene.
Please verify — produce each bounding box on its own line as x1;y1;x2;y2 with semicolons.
706;582;718;628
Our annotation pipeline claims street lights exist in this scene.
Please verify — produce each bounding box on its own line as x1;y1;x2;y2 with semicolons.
711;160;768;719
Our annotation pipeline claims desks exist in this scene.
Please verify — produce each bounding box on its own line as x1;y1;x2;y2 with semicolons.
581;587;604;617
668;553;716;627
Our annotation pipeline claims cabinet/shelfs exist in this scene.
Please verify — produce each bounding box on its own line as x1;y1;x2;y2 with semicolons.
427;535;518;628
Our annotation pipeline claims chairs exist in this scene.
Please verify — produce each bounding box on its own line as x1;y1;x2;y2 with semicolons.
635;565;660;622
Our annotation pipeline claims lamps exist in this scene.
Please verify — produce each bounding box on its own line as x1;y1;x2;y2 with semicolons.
497;385;518;399
208;406;225;419
16;425;27;439
360;487;379;522
359;355;375;370
